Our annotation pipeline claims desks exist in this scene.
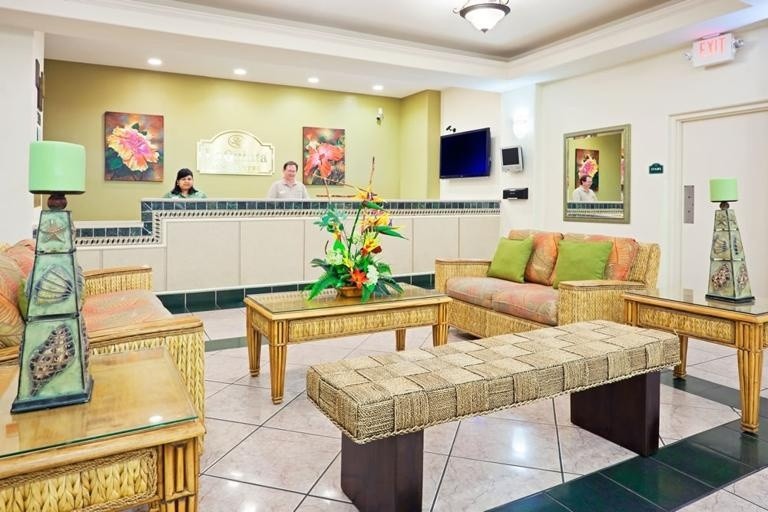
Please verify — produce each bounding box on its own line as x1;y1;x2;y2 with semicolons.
0;345;206;512
619;286;768;437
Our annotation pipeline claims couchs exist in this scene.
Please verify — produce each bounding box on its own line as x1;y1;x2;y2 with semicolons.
432;229;662;340
0;235;205;427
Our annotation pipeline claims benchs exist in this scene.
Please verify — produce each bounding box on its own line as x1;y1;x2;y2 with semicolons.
304;317;685;512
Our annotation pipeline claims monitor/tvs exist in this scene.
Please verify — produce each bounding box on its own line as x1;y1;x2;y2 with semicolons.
502;145;522;174
441;126;491;179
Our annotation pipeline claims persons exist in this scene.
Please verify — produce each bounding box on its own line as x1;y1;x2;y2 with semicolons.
160;169;208;199
571;175;600;203
265;162;310;200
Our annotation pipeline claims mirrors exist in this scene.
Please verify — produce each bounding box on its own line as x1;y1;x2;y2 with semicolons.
561;124;631;224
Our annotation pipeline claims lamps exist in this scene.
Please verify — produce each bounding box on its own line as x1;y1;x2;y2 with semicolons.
705;178;756;305
450;1;512;39
10;141;95;416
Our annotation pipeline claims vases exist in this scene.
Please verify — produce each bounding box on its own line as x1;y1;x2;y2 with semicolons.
339;284;367;298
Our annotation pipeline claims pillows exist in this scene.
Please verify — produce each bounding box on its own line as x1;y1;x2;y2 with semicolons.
548;236;617;292
550;232;638;282
0;237;45;353
486;236;535;284
506;229;564;286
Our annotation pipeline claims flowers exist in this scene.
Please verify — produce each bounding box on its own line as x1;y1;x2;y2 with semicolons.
104;122;163;179
304;130;345;184
579;156;598;185
300;154;409;303
